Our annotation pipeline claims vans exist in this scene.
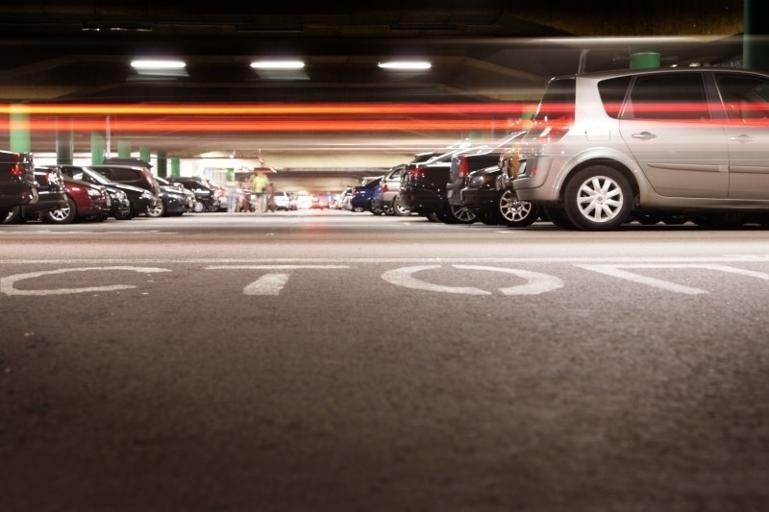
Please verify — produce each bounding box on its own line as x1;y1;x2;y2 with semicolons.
513;65;769;229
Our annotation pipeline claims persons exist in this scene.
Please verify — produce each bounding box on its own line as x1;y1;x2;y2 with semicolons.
225;168;275;214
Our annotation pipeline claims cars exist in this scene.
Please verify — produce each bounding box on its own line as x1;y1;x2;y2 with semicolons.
459;163;541;227
329;128;533;225
0;149;255;224
272;191;296;210
